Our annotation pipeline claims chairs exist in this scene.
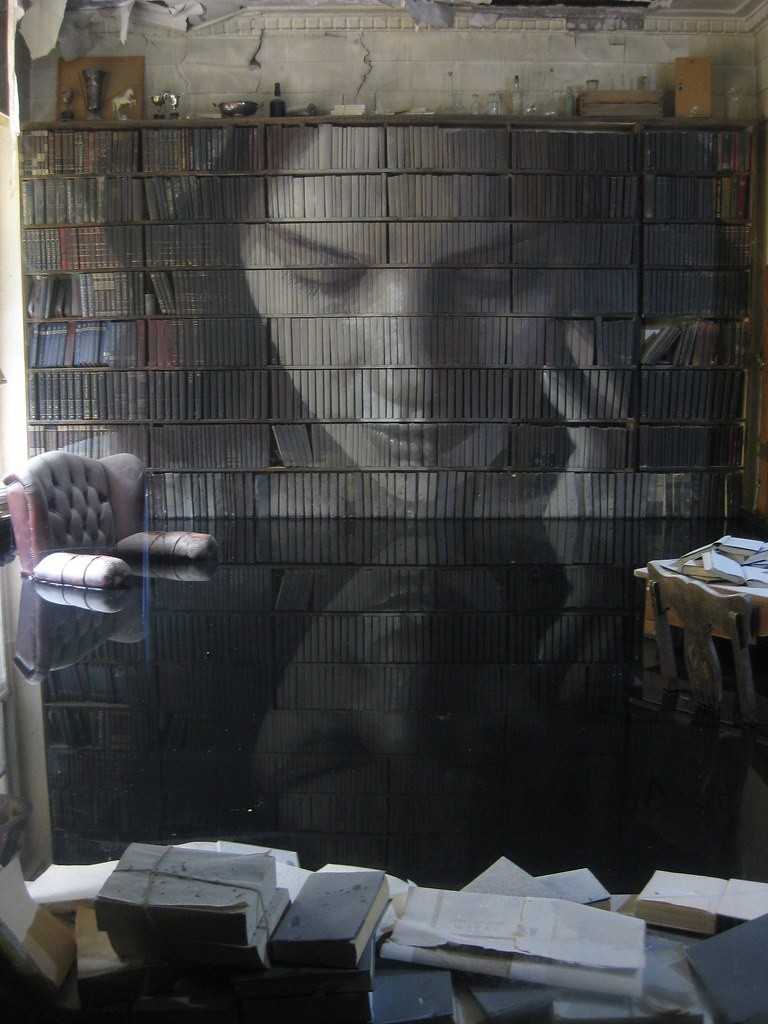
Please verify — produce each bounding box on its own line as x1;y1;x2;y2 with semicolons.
645;559;761;724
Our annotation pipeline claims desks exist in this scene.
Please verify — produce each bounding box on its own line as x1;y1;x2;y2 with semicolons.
633;567;768;637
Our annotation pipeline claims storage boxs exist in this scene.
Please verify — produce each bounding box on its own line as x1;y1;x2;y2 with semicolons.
676;56;711;117
575;83;663;117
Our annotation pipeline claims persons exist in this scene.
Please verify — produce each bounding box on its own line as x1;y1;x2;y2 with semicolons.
172;517;625;899
149;123;752;518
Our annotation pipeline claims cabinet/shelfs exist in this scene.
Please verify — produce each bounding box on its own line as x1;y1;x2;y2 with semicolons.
40;520;746;891
17;115;768;520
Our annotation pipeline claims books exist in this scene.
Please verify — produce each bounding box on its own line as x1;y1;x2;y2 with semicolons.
1;129;768;1024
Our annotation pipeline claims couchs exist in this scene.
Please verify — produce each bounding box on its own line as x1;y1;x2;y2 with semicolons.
11;574;221;683
3;451;219;588
